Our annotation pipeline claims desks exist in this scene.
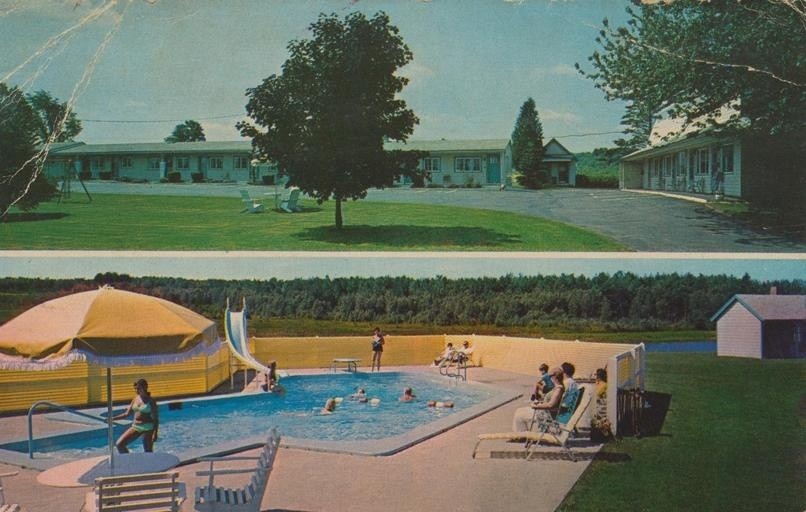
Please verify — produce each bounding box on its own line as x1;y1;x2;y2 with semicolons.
331;358;364;374
263;192;283;211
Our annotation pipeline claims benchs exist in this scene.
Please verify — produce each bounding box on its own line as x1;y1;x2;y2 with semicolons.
93;471;189;511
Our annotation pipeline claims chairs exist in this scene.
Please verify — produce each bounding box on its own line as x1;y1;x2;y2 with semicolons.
279;188;300;214
522;382;593;448
471;392;592;463
0;470;22;512
192;424;282;510
238;188;265;214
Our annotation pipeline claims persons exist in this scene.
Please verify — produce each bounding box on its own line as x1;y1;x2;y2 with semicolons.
454;340;475;368
370;327;385;373
710;170;726;195
588;367;608;419
105;377;158;454
532;361;555;398
507;366;564;443
435;342;455;371
262;359;286;396
558;361;578;414
320;386;415;415
